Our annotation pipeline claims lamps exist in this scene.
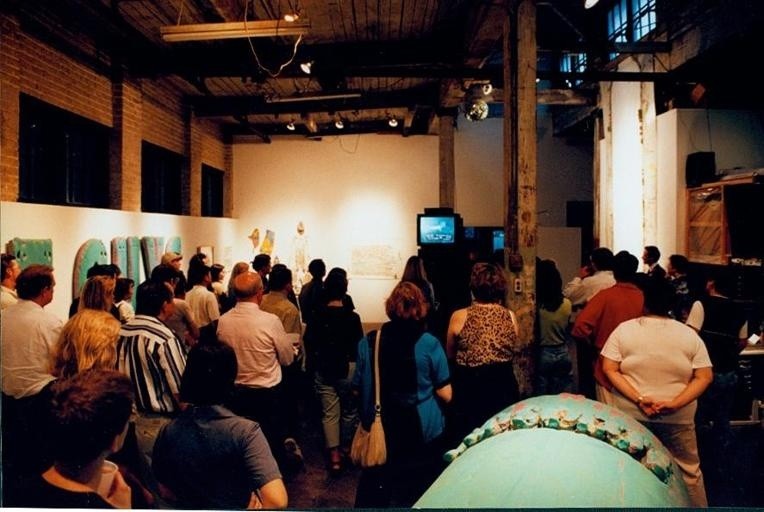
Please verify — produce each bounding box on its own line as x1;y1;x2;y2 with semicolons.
389;116;398;126
335;118;344;129
287;118;296;131
301;60;313;74
283;9;305;22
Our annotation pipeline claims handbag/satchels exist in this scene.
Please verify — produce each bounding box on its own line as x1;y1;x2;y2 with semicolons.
350;414;387;467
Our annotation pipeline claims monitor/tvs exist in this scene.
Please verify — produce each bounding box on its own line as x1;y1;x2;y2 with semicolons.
490;228;505;252
416;213;463;249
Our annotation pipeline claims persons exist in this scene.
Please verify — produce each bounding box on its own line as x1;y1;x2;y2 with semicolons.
392;255;436;319
448;246;749;510
1;252;353;510
355;281;453;511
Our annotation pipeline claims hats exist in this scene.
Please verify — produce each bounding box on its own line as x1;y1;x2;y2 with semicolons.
162;252;182;264
87;264;114;277
151;264;176;282
